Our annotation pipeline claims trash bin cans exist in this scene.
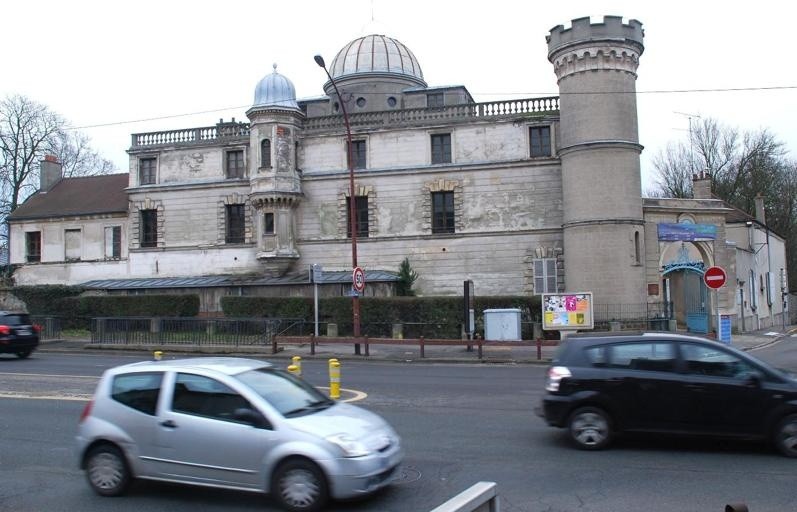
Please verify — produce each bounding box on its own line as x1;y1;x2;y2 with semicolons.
650;319;670;331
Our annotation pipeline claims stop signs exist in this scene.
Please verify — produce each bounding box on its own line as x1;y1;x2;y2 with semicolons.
701;266;728;290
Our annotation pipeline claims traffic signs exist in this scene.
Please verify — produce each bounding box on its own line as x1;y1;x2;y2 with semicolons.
353;267;366;291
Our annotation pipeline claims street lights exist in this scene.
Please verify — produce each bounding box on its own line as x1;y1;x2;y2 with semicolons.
313;53;362;354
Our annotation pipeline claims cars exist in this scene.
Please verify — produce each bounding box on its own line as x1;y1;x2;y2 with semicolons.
533;331;796;458
0;310;39;361
75;355;404;511
